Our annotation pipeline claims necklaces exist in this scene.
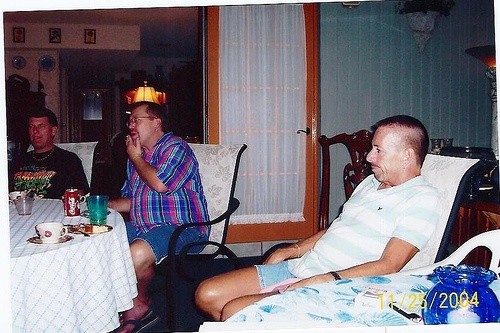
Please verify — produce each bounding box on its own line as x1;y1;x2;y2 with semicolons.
32;145;55;162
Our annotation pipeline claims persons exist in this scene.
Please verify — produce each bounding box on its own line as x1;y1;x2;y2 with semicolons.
193;114;441;323
106;101;211;333
6;108;91;200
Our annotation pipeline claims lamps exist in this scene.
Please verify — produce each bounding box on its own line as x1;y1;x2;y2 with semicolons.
133;81;160;104
464;43;500;161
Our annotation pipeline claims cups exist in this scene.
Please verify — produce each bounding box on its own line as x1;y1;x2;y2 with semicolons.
87;195;109;225
62;194;86;205
8;191;24;204
35;222;68;243
12;198;35;215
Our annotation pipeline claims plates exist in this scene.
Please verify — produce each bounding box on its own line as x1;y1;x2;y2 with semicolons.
38;55;56;72
63;223;113;235
12;56;26;70
26;235;74;246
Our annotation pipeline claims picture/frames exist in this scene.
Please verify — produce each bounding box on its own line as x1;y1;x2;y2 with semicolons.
13;26;25;43
84;28;96;44
48;27;62;44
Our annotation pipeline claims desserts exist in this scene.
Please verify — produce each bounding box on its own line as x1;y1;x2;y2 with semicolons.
85;224;109;233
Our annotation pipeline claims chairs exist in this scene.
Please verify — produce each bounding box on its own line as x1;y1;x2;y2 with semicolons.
161;143;248;333
404;151;483;270
27;141;98;188
317;128;375;233
383;227;500;280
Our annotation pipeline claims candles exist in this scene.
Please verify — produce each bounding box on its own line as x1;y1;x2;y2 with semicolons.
446;300;480;325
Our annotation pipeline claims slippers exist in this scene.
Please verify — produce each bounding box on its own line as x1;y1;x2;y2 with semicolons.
117;311;161;333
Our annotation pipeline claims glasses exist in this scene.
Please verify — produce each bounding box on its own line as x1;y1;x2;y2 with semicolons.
125;116;157;127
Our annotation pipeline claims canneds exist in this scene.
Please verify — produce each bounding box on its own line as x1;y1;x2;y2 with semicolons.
64;187;80;216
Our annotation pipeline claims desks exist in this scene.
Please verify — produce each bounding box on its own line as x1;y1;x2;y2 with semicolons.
222;270;500;333
444;198;500;267
10;199;138;333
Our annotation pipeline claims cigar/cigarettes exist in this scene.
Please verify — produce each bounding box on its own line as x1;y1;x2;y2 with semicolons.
125;135;129;138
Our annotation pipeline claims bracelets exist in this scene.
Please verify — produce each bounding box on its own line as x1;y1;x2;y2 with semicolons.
293;242;302;258
132;154;142;163
328;272;342;281
